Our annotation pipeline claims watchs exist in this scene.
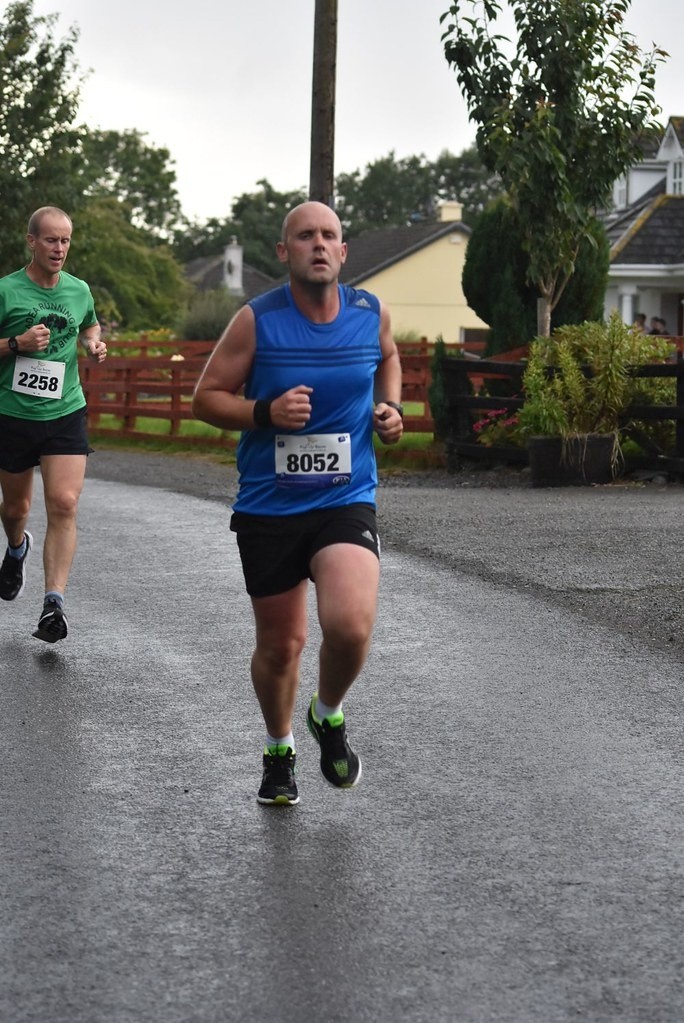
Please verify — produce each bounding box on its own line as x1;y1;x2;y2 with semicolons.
8;335;23;355
376;400;404;420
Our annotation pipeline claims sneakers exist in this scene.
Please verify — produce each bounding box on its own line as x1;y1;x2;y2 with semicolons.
304;691;362;790
255;744;301;806
31;598;69;644
0;530;33;602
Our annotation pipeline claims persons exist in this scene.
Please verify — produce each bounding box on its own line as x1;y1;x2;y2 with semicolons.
187;201;406;806
1;203;107;644
627;312;671;335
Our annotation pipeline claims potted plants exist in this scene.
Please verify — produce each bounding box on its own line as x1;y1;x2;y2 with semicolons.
513;306;682;488
